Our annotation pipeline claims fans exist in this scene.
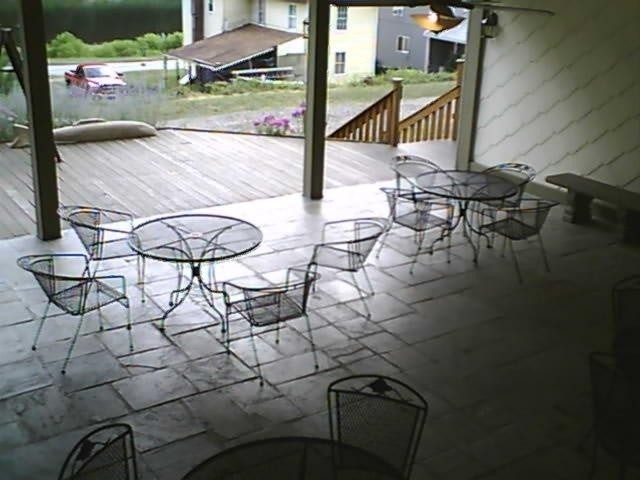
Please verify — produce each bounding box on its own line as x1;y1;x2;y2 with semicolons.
324;0;557;36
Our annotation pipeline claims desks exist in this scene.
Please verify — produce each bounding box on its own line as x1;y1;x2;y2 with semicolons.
180;435;406;479
128;214;263;333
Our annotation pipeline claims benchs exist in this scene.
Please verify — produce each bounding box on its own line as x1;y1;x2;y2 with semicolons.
544;172;640;241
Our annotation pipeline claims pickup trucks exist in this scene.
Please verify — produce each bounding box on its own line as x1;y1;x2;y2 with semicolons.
64;62;128;102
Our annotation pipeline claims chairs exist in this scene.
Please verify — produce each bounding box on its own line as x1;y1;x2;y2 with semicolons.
303;217;391;316
223;267;319;387
56;423;141;480
376;155;536;276
57;205;145;303
325;370;430;480
474;198;561;284
16;253;134;375
611;273;640;357
584;347;640;479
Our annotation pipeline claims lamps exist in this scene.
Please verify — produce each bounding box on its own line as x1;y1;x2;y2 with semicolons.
408;10;465;35
481;10;500;38
